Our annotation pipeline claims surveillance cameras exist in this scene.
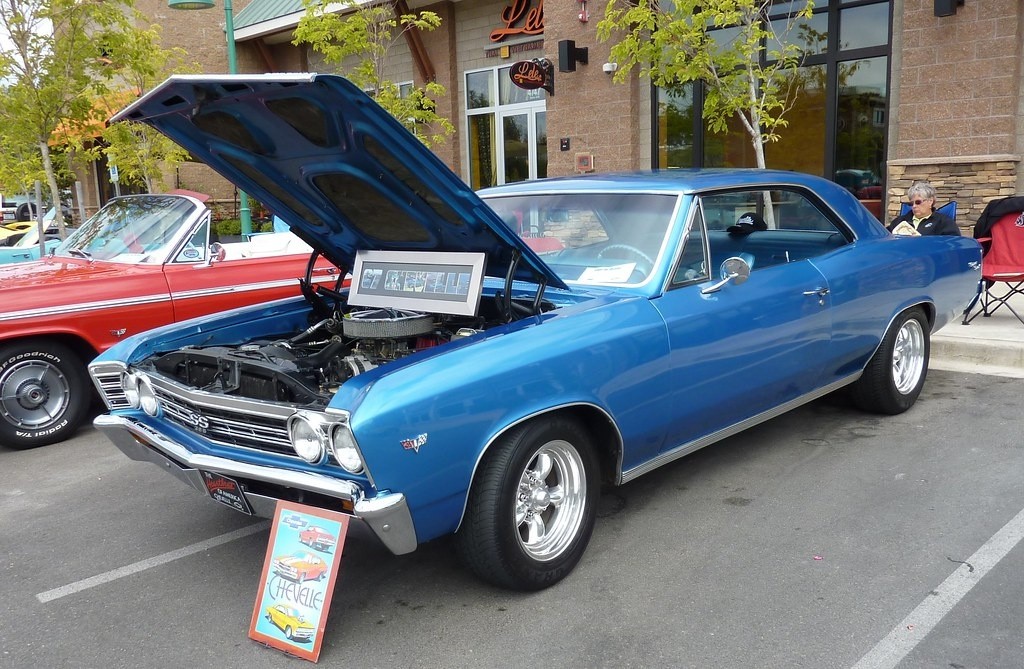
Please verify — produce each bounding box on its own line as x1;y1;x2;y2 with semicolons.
602;63;613;74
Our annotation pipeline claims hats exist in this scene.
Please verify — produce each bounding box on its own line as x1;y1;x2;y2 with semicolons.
727;212;767;234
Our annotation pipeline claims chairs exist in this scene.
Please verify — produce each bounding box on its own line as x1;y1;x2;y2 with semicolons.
962;197;1024;325
900;201;957;221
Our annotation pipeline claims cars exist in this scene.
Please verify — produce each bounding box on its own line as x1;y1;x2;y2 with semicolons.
86;73;985;595
0;193;162;276
298;525;335;550
274;552;330;584
0;188;564;450
266;602;314;640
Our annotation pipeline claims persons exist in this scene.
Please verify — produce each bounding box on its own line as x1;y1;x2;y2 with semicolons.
886;182;962;235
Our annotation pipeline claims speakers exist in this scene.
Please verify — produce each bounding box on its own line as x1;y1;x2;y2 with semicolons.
559;40;576;72
934;0;955;17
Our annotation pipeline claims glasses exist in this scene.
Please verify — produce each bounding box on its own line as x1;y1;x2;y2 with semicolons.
908;199;926;206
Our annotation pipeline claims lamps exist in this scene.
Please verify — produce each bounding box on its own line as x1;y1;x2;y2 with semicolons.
558;40;588;73
413;92;436;123
934;0;965;17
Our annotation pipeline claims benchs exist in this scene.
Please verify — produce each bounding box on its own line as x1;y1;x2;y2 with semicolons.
680;229;849;273
